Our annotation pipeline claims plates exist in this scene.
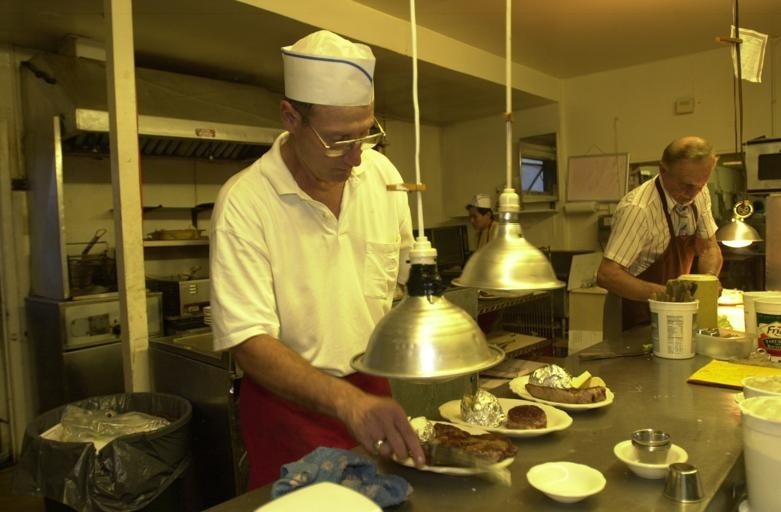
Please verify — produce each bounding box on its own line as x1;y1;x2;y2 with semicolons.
438;398;574;439
253;481;384;512
508;374;614;411
391;420;515;477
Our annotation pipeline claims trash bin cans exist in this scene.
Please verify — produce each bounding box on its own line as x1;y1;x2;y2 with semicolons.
26;392;193;512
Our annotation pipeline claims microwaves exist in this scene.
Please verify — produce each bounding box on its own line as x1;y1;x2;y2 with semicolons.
745;141;781;191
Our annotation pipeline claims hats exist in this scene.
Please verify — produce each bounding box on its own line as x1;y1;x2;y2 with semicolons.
468;195;490;209
280;29;376;106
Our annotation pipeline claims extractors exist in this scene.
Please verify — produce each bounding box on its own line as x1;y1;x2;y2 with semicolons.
17;54;288;161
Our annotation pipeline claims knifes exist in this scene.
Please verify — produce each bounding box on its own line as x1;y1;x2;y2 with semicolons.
578;351;649;361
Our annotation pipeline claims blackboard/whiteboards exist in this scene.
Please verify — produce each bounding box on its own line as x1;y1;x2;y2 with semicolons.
566;152;630;202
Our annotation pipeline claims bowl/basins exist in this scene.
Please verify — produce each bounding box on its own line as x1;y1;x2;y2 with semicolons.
662;463;706;504
613;439;689;480
526;461;607;504
630;428;672;464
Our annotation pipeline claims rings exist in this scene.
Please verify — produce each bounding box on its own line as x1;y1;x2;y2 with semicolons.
373;440;384;456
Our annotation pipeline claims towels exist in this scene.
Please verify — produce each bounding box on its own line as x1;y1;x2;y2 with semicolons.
272;446;413;510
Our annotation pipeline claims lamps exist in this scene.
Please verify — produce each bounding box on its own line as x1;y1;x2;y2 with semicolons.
716;0;764;250
350;0;505;383
451;0;565;301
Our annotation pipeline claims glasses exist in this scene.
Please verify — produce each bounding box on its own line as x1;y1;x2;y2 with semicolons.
287;103;386;158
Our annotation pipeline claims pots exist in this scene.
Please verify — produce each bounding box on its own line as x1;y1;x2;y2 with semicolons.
147;229;208;240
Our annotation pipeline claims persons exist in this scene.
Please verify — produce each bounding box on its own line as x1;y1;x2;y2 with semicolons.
465;193;502;329
597;135;724;331
208;29;427;492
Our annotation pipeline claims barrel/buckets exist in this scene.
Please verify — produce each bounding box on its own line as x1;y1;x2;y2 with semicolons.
646;299;701;360
737;397;781;511
743;377;780;397
740;292;780;335
753;297;781;357
